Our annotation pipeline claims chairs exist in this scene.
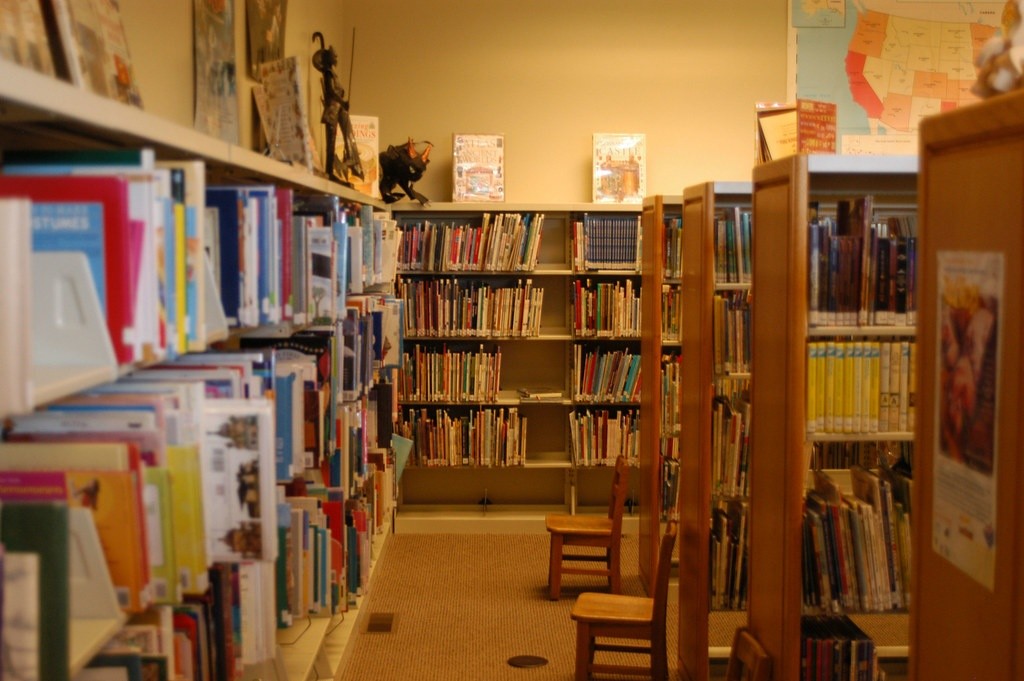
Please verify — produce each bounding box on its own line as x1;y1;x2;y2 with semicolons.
724;626;772;681
570;520;676;681
545;455;629;601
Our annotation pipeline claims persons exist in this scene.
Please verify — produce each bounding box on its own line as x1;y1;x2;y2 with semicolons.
323;45;350;177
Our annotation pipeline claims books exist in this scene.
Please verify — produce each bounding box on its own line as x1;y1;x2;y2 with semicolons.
0;0;1024;681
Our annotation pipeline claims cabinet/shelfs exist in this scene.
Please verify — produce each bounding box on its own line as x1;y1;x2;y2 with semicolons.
0;0;1024;681
